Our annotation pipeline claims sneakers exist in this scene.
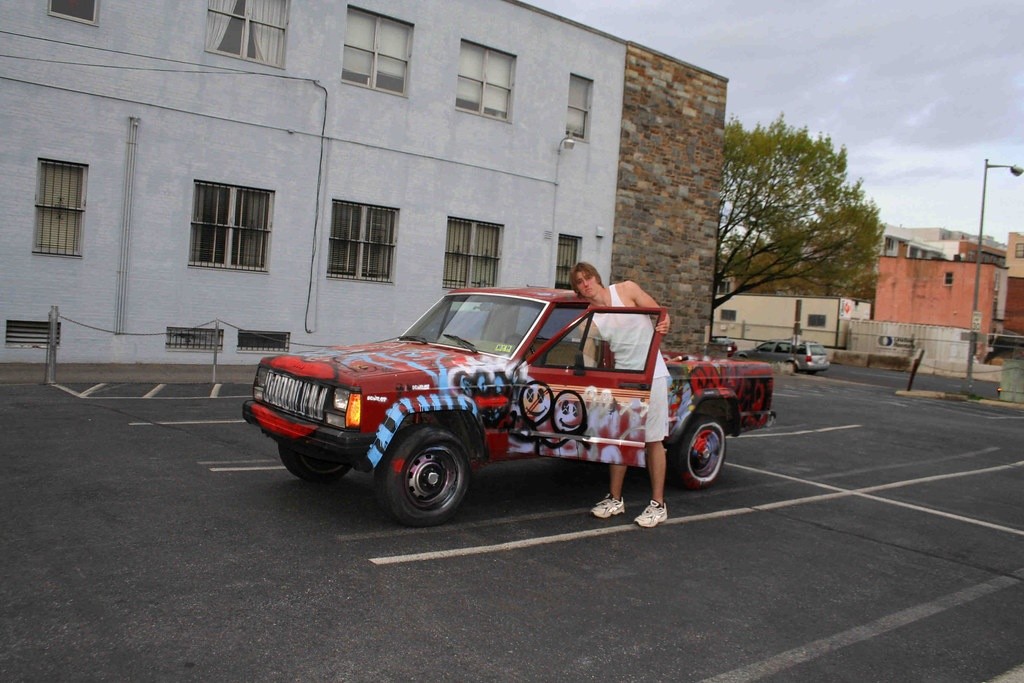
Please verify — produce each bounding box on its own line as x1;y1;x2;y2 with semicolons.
590;493;625;519
634;500;667;527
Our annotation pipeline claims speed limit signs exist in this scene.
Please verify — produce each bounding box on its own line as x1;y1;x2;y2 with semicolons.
971;311;982;333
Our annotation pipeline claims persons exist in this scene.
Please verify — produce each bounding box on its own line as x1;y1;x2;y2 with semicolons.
568;262;669;528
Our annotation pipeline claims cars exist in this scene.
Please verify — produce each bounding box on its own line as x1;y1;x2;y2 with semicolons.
734;337;830;375
712;333;738;357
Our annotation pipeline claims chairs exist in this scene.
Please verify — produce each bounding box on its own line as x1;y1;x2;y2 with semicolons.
527;340;578;366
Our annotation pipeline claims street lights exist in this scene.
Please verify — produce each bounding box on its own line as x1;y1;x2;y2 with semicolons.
966;161;1024;381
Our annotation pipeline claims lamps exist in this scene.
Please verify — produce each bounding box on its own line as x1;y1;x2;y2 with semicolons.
557;129;574;155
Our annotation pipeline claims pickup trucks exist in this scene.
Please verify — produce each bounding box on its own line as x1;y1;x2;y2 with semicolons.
243;285;780;528
